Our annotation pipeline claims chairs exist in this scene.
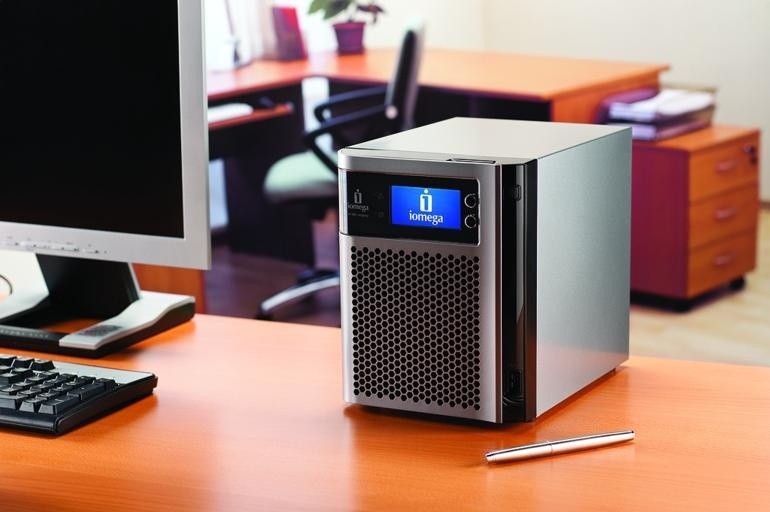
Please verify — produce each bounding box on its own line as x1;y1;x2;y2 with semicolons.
259;22;422;320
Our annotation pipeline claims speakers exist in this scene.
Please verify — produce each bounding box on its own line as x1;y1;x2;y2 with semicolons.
336;116;633;427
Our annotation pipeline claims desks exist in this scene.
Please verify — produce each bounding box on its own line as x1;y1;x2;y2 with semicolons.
0;316;770;512
208;46;669;184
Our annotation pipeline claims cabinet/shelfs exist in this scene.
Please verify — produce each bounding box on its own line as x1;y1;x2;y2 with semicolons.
631;123;760;298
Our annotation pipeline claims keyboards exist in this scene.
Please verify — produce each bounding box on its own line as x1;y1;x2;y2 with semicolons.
0;352;159;438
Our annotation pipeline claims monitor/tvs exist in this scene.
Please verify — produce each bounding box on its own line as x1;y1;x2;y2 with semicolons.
0;0;213;359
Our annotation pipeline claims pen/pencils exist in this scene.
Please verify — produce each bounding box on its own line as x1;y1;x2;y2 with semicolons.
484;431;635;462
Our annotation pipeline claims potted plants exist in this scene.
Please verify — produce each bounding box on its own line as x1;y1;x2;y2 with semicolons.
306;0;382;54
